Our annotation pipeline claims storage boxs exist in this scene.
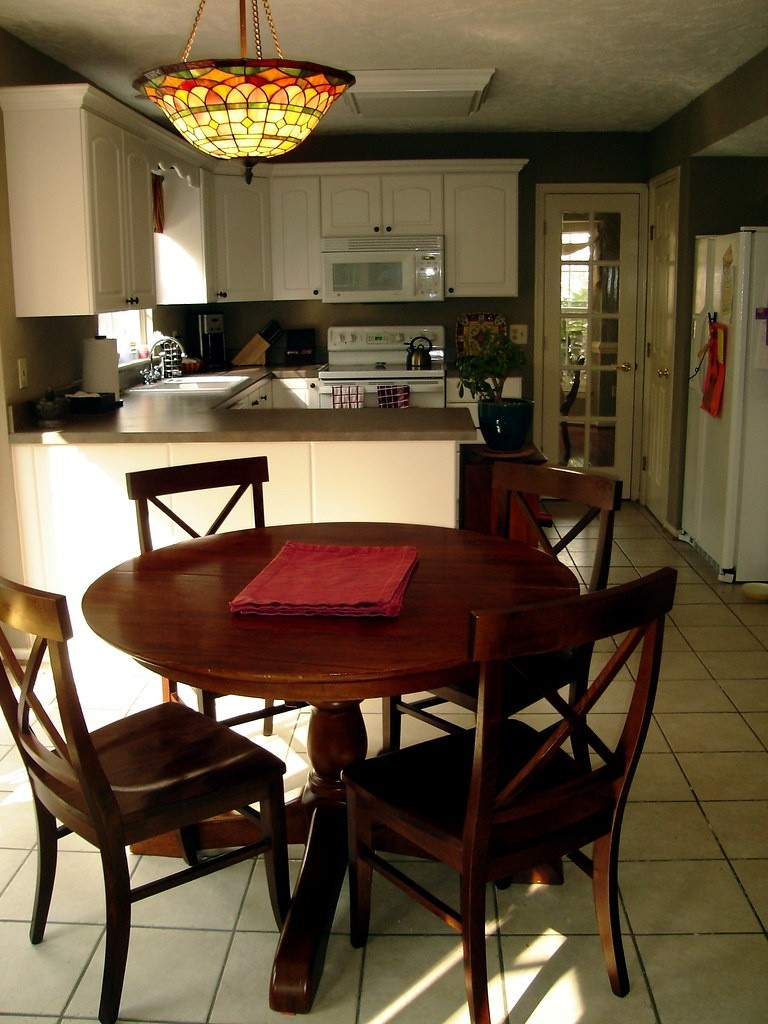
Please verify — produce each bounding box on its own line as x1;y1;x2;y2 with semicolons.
284;329;317;366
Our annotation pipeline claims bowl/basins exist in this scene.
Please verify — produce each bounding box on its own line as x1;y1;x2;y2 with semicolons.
741;582;768;602
182;360;202;375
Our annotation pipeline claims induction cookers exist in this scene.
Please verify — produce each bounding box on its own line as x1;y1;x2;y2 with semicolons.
318;326;447;379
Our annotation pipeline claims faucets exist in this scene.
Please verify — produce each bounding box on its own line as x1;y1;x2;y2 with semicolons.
149;335;187;380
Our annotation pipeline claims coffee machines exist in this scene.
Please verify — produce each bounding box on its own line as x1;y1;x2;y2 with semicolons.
186;312;228;369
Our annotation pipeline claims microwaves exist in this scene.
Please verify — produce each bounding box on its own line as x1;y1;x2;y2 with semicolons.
321;237;445;304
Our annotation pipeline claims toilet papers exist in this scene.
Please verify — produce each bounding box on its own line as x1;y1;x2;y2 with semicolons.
82;337;120;401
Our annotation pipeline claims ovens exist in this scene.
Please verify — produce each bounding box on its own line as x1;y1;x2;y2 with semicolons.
319;380;445;408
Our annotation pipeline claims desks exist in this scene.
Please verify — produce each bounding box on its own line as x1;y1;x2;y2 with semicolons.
82;521;580;1013
471;445;537;526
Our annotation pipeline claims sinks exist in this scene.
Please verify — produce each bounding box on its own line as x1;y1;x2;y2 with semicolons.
134;382;240;389
162;375;246;383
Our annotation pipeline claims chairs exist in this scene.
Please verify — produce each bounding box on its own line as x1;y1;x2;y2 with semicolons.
126;456;311;737
382;461;623;771
560;355;585;465
0;577;290;1024
342;565;678;1023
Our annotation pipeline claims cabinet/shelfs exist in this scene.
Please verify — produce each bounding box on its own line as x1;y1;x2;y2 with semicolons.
320;160;443;237
446;378;522;444
272;378;320;409
248;380;272;409
0;84;157;318
443;159;529;297
151;121;272;304
270;163;322;300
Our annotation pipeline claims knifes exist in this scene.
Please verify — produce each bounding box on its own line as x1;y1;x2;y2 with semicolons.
260;318;285;344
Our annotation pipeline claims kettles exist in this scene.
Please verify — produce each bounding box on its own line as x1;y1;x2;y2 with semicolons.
406;336;432;366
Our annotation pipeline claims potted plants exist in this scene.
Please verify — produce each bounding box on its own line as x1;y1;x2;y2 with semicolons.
455;329;534;450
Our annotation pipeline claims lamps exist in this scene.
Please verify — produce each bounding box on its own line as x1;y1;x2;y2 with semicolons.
337;68;495;118
132;0;356;186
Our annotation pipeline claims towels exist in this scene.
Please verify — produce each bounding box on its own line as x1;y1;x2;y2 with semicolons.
331;386;365;408
377;384;410;408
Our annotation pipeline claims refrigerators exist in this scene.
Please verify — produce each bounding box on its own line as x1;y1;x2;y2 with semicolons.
680;224;768;585
